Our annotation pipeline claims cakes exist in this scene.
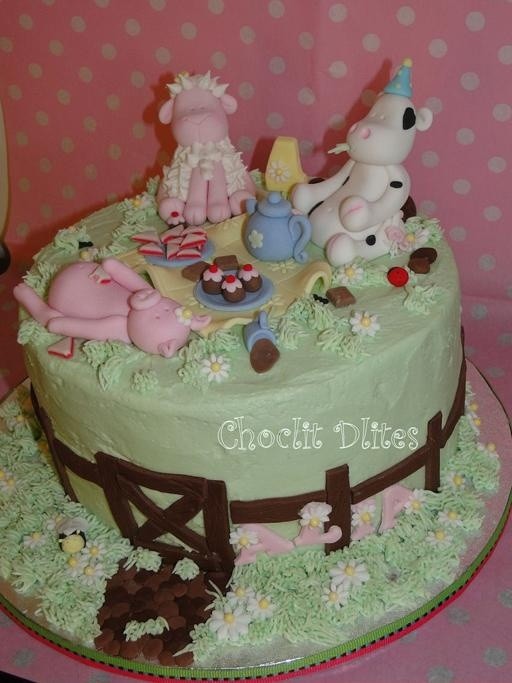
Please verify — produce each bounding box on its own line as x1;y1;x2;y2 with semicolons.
1;57;510;683
